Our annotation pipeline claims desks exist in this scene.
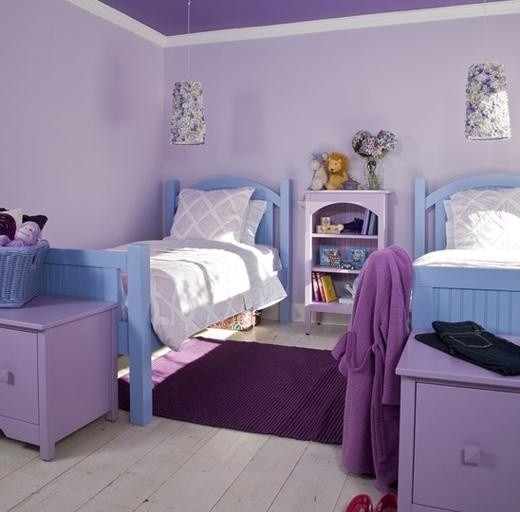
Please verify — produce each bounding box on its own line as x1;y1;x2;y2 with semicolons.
395;330;520;512
0;295;119;461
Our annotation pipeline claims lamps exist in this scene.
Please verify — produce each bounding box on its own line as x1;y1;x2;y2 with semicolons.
464;0;511;141
170;2;204;146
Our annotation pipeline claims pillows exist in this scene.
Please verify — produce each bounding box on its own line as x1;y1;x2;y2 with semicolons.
443;187;520;250
163;187;255;245
240;200;267;246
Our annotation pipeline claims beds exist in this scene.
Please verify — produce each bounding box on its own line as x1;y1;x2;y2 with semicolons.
32;177;292;426
408;175;520;337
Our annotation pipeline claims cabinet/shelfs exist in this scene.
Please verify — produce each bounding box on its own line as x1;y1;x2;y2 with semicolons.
301;189;391;336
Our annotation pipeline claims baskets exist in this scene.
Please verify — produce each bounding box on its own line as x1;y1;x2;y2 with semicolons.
1;240;48;309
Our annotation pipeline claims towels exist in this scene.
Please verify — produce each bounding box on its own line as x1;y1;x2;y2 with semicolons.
330;246;412;494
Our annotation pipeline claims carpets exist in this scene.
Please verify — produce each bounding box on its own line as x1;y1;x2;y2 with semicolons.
118;338;347;446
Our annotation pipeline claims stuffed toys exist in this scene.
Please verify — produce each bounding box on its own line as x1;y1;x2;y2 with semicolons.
323;152;351;190
297;152;328;206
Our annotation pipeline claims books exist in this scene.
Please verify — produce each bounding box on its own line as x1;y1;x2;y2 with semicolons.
368;213;376;235
361;209;370;235
312;272;354;304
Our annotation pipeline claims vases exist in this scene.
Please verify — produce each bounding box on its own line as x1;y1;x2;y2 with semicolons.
367;162;383;192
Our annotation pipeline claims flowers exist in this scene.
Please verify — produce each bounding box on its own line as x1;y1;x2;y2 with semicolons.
353;130;397;171
